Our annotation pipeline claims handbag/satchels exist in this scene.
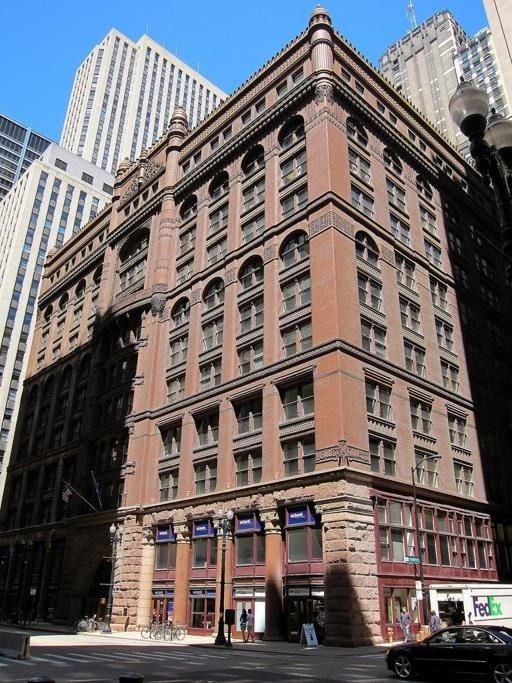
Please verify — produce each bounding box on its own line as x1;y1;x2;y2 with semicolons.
240;618;244;625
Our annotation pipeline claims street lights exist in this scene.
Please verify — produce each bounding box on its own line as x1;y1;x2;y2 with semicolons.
448;74;512;239
12;527;36;626
101;524;125;635
408;455;443;625
213;508;235;648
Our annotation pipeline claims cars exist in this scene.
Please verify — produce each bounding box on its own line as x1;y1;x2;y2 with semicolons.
386;624;512;683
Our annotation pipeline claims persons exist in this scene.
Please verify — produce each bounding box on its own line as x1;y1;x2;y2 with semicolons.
238;608;248;643
399;606;411;643
428;608;442;632
245;607;256;643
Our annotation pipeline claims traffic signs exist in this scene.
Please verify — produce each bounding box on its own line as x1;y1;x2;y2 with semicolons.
409;557;421;563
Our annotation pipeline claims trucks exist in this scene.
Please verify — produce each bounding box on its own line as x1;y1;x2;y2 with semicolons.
427;581;512;644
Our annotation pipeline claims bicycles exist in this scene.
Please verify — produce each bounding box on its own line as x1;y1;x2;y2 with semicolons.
2;607;36;627
64;612;103;633
140;615;187;641
